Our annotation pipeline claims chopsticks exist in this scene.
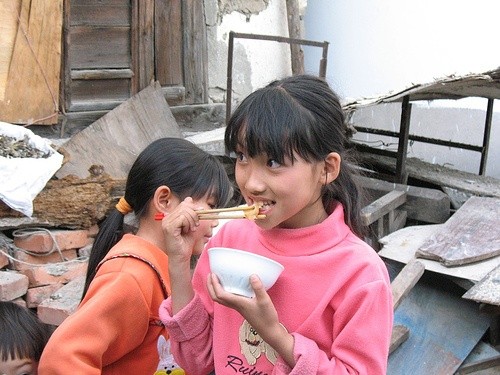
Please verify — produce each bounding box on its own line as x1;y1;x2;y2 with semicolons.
154;205;268;219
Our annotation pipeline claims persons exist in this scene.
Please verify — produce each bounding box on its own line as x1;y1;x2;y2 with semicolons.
38;137;232;375
0;302;52;375
159;73;394;375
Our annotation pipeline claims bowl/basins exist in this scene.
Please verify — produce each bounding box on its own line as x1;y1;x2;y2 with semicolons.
207;248;285;298
